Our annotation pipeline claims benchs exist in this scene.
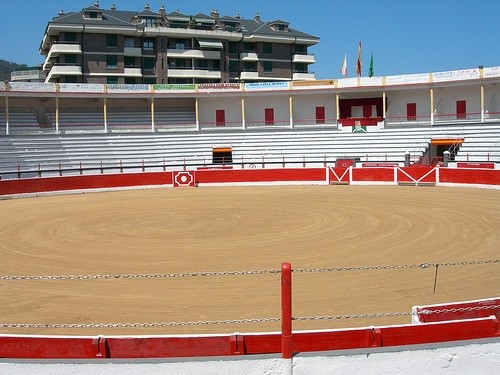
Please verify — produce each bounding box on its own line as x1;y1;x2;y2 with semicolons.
2;102;499;180
